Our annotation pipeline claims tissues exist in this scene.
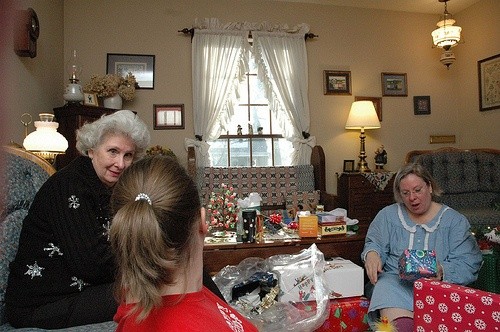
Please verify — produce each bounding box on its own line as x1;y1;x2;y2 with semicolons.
316;208;346;223
235;191;263;242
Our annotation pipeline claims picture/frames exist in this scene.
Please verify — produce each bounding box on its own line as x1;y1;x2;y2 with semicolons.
323;69;431;121
106;53;185;130
477;53;500;112
343;159;354;172
84;92;98;106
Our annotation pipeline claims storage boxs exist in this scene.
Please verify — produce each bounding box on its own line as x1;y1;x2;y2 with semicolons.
398;248;437;282
273;256;370;332
412;277;500;332
297;212;347;237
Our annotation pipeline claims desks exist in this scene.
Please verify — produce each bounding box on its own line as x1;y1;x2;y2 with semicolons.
203;229;366;279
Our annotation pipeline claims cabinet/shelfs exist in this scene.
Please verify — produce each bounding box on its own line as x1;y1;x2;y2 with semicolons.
53;104;137;172
336;172;397;234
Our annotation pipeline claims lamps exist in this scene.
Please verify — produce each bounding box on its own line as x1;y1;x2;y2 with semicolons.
344;101;381;172
431;0;462;71
61;50;84;105
23;113;69;166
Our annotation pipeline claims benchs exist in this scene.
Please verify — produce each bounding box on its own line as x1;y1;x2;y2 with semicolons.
0;144;119;332
186;146;326;226
404;147;500;232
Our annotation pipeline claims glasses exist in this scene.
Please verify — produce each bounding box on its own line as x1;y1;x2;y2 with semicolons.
401;183;427;197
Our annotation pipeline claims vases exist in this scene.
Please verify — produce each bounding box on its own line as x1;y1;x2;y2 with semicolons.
103;94;123;109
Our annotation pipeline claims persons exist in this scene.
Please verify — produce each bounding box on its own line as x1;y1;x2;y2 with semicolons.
4;110;151;330
361;163;482;332
108;157;259;332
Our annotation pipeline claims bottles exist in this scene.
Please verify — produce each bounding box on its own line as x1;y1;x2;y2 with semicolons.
242;210;257;244
316;205;325;214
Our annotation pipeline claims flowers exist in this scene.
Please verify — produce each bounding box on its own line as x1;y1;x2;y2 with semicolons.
83;71;138;101
